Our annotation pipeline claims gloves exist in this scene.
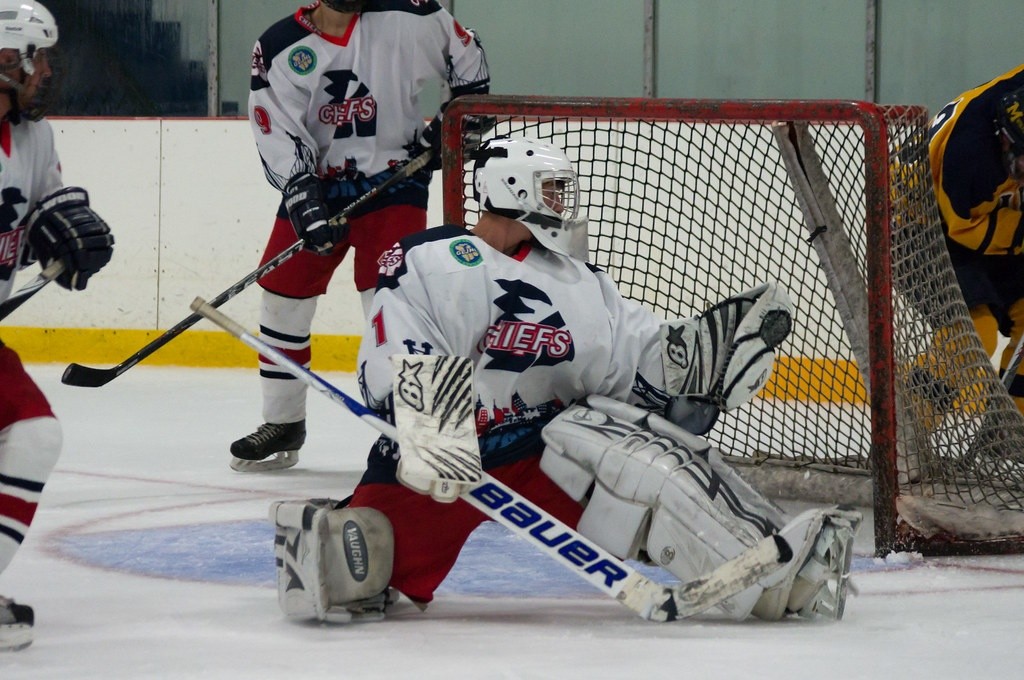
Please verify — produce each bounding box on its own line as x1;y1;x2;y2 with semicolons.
26;181;117;293
282;176;353;253
409;103;495;172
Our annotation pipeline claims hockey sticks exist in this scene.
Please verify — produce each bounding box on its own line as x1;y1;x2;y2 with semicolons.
60;145;438;388
190;295;793;623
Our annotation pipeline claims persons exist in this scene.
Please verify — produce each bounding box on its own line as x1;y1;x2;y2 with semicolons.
0;1;115;652
334;138;843;618
230;0;490;472
888;63;1023;483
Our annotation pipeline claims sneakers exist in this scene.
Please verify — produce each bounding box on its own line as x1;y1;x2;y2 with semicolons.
0;589;36;654
881;419;940;485
755;513;848;617
227;419;309;475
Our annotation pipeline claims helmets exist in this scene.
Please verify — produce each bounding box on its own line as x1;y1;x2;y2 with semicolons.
475;138;590;265
0;1;59;55
